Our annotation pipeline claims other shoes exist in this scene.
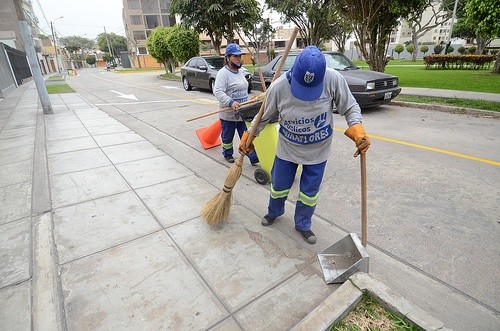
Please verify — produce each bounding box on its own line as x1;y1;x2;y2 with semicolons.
253;162;260;166
226;155;234;163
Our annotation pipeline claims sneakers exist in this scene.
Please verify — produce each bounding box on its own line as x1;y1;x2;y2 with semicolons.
262;212;284;225
300;229;316;243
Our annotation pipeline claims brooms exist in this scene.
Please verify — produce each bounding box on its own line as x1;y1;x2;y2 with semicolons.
185;91;266;123
202;26;300;228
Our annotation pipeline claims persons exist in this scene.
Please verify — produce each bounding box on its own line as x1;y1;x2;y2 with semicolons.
213;43;261;167
238;46;370;244
106;61;110;71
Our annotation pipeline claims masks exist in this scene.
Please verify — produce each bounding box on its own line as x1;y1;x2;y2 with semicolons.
228;57;242;69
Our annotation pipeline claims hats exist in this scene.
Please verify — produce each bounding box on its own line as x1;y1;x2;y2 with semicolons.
291;46;326;101
225;44;246;57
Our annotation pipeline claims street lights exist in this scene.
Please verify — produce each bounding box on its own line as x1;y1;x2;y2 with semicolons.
50;16;64;73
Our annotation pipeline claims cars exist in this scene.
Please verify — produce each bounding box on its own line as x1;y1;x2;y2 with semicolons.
180;55;254;95
250;50;402;114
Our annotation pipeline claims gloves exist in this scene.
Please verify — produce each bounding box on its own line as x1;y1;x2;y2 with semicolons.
344;123;370;157
238;131;256;155
